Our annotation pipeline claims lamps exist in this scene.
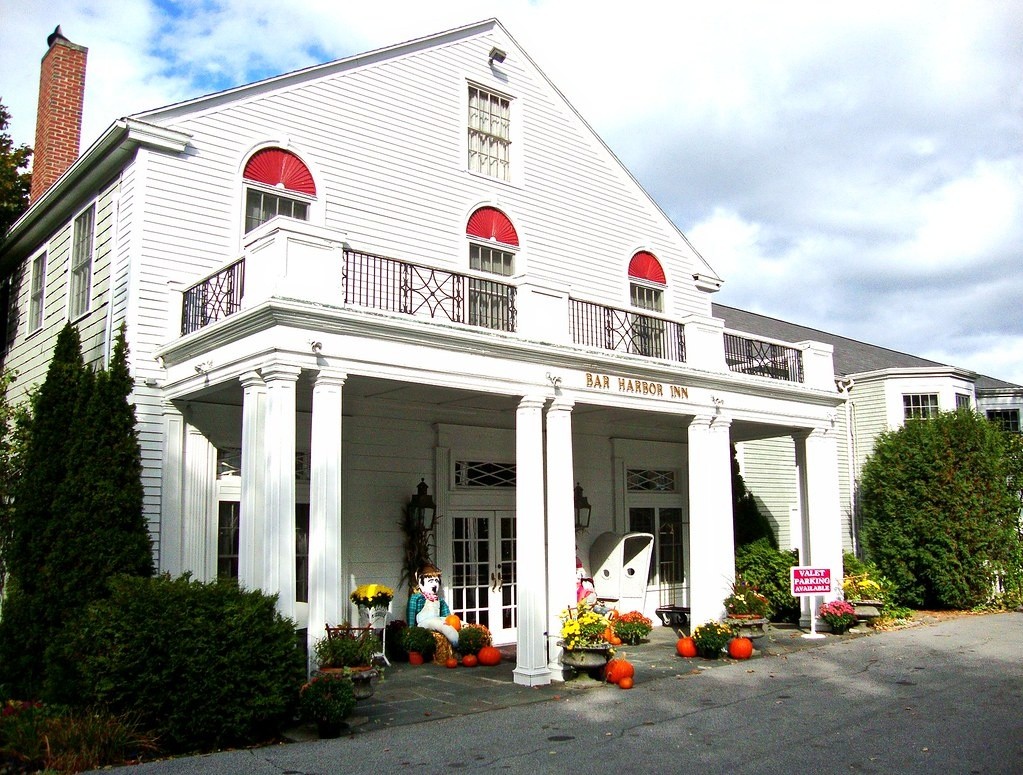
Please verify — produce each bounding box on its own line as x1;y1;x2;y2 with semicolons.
547;371;563;386
195;360;214;374
488;46;507;66
310;342;323;354
708;397;724;411
409;475;436;531
574;481;592;532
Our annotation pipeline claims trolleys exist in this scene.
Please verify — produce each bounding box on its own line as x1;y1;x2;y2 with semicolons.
656;561;690;627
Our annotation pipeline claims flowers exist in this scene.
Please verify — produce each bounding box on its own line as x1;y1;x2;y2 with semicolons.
299;666;358;723
555;600;654;655
348;583;394;608
693;571;779;649
816;578;880;627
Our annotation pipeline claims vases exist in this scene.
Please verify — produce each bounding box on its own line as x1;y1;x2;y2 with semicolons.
701;648;720;660
723;610;770;642
555;639;610;689
627;634;640;647
359;604;388;619
832;622;844;634
320;665;378;726
846;599;883;634
318;719;341;739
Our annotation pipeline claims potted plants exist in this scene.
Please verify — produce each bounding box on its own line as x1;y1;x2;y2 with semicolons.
313;622;437;667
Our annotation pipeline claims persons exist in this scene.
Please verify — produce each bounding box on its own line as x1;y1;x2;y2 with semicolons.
576;556;610;615
407;563;468;647
